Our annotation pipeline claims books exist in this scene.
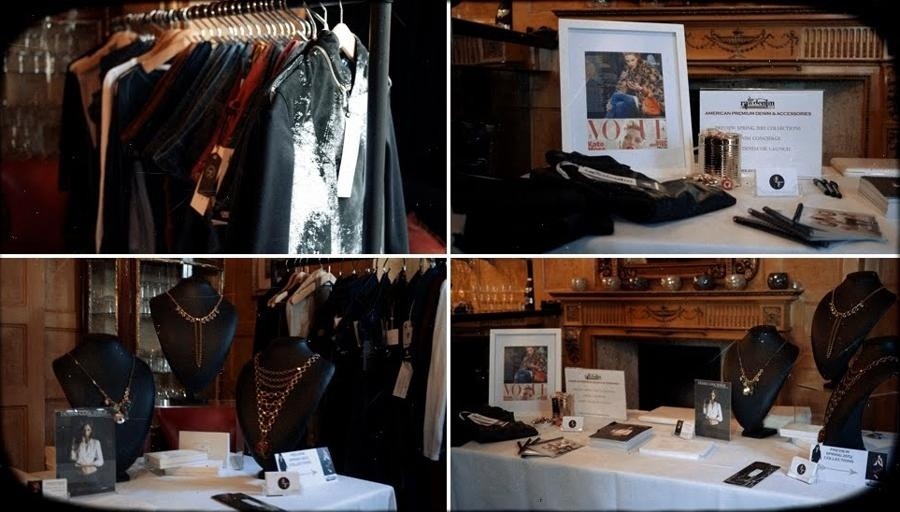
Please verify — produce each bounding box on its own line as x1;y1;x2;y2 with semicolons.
733;202;887;249
143;429;231;475
860;177;900;216
767;402;823;453
515;436;584;460
638;436;716;460
589;421;652;452
830;157;900;178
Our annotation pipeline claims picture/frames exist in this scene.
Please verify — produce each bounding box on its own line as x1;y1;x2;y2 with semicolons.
487;327;562;418
556;18;695;182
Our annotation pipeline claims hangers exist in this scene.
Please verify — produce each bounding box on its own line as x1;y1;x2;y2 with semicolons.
103;0;358;61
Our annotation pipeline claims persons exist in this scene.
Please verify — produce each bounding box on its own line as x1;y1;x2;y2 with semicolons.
618;121;648;150
704;389;723;433
873;453;884;479
811;445;822;462
514;347;535;383
604;52;665;117
529;347;547;383
71;421;105;485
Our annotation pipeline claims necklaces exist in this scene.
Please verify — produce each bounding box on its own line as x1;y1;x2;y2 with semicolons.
825;287;886;359
737;339;786;398
817;349;898;444
166;290;224;369
254;352;320;455
67;351;137;426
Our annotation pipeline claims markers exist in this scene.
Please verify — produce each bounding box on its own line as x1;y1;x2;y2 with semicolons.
733;203;830;247
517;437;541;458
813;179;842;198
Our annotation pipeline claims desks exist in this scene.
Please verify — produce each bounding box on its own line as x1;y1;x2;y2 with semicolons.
65;473;397;512
451;414;856;512
